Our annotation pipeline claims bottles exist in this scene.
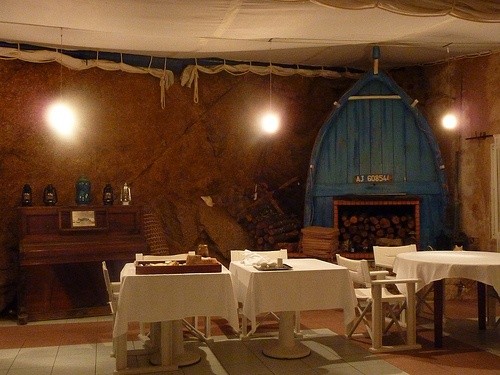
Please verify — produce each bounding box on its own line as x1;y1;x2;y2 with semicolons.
278;258;283;268
103;183;113;205
186;244;217;265
43;184;57;206
75;175;90;205
120;183;131;206
22;184;32;206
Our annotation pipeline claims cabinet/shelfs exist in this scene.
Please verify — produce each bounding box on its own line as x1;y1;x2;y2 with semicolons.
13;202;147;324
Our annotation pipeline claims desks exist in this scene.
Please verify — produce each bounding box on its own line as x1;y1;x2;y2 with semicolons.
392;250;500;349
112;258;239;366
229;258;359;359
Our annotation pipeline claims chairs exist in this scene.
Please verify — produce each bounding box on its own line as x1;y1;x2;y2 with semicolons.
101;260;151;358
231;249;304;341
136;250;215;344
336;254;421;353
373;244;433;326
